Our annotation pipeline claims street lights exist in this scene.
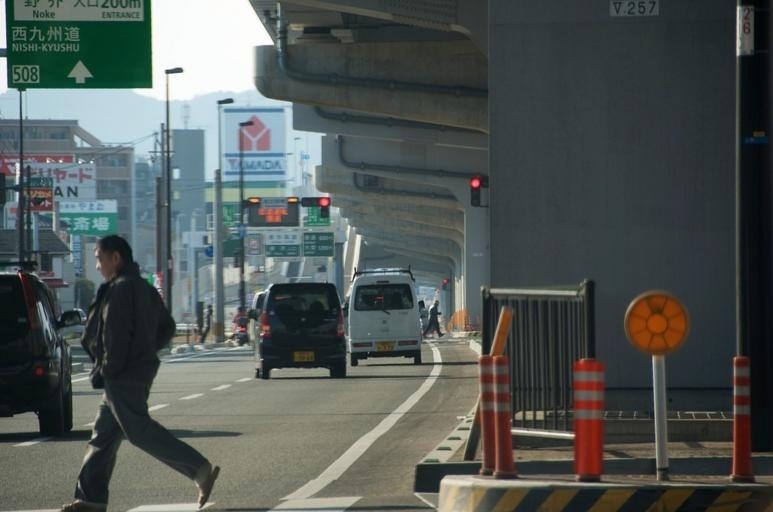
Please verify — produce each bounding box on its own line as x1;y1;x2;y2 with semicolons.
165;67;253;315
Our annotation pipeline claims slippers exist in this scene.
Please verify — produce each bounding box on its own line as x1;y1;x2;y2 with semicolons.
62;500;106;512
198;466;221;510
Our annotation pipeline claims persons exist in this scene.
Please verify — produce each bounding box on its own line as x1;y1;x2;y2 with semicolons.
61;233;220;512
229;307;242;339
422;300;444;338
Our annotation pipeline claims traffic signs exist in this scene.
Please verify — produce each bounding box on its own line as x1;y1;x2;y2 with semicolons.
6;0;151;87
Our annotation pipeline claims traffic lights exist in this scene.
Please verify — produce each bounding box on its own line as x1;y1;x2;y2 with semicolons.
470;177;481;206
300;197;331;207
440;279;449;290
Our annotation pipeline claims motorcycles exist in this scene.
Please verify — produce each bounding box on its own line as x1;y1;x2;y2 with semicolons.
231;312;250;346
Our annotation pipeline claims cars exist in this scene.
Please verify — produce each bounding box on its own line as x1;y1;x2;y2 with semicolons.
0;270;87;436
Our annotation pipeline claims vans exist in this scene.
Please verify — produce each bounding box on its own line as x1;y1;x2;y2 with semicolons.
248;264;425;379
